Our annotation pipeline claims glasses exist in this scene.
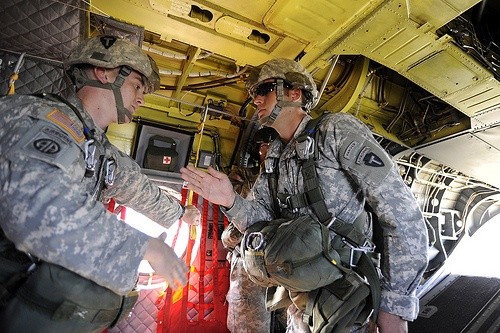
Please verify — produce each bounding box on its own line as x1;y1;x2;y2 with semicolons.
255;81;293;96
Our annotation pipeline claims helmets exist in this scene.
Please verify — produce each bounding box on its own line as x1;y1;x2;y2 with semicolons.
245;58;318;108
63;35;160;95
251;126;279;162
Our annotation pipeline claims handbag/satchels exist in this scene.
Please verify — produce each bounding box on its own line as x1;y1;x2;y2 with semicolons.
0;262;139;333
240;215;367;293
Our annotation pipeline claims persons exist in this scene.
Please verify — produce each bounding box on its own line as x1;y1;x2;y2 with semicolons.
179;58;430;333
0;39;204;333
